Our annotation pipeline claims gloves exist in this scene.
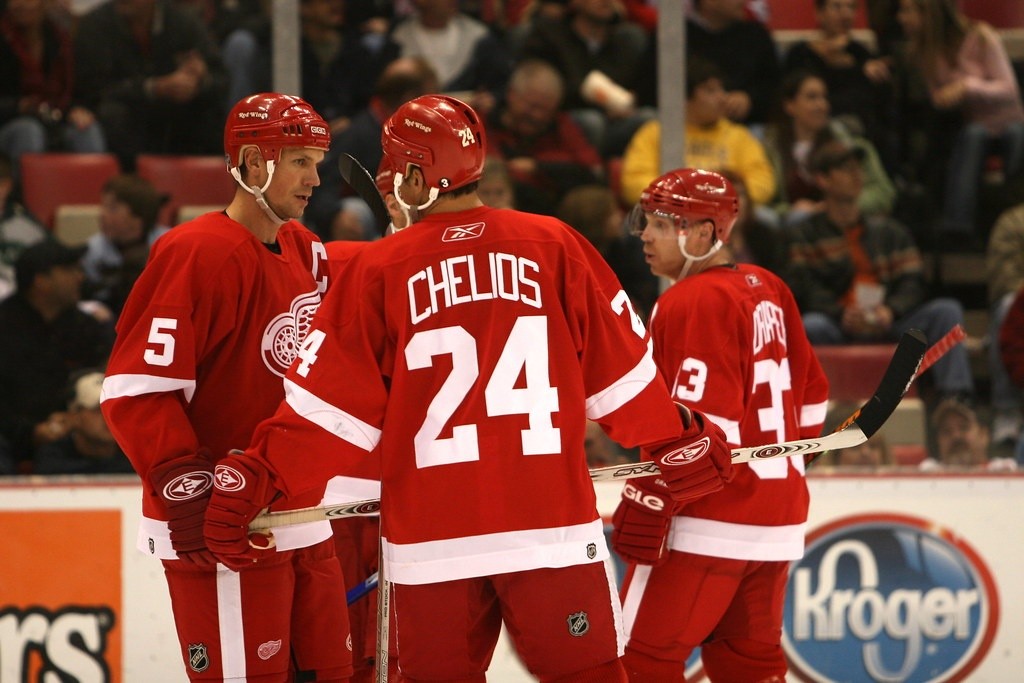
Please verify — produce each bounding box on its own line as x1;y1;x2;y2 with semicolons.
146;452;216;564
610;471;679;563
203;450;277;568
645;406;737;502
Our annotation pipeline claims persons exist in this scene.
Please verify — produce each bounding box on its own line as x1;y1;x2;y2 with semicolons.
99;93;436;683
617;168;830;683
206;94;738;683
0;0;1024;475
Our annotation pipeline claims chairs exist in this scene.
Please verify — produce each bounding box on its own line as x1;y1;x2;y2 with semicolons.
0;0;1024;475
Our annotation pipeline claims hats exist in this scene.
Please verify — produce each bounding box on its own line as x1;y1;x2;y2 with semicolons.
932;397;994;431
15;241;88;281
806;140;866;173
66;372;105;416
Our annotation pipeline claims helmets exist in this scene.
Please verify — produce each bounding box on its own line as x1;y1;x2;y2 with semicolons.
225;92;330;168
382;94;486;192
642;168;740;242
376;158;400;197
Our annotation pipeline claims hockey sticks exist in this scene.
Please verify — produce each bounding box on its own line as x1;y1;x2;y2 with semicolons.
376;577;392;683
248;326;931;532
342;570;380;607
802;325;972;475
